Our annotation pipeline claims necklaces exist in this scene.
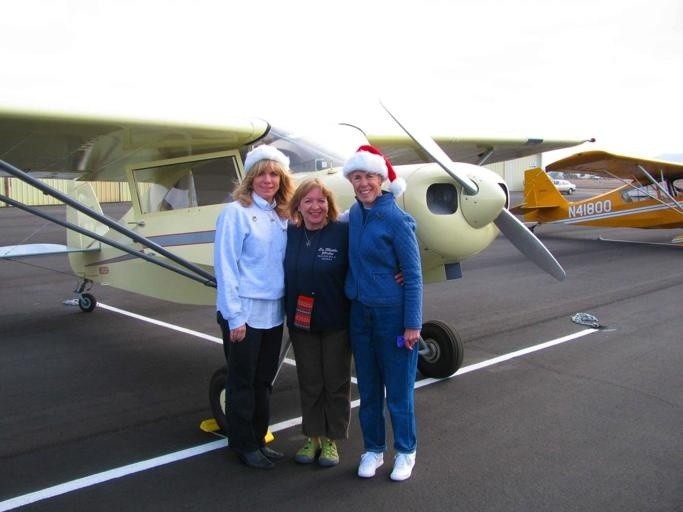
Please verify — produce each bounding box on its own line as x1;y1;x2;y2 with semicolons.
302;220;330;247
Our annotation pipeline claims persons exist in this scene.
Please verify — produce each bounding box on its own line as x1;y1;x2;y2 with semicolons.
213;144;350;470
279;178;352;467
342;145;426;482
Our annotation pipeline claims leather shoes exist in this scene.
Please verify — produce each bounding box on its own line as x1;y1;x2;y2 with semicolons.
260;446;283;459
238;449;274;470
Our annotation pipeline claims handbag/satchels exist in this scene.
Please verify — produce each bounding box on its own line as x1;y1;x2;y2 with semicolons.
293;294;314;329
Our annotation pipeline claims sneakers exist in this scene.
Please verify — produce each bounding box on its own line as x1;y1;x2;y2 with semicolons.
294;438;321;463
318;439;339;466
390;450;416;481
358;452;383;478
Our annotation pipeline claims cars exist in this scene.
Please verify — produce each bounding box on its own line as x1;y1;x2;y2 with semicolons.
551;178;575;196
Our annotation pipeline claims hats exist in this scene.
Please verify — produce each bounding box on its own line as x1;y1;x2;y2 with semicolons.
244;145;290;173
343;145;406;197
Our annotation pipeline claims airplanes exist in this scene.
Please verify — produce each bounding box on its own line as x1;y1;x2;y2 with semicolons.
507;148;683;247
0;97;594;435
